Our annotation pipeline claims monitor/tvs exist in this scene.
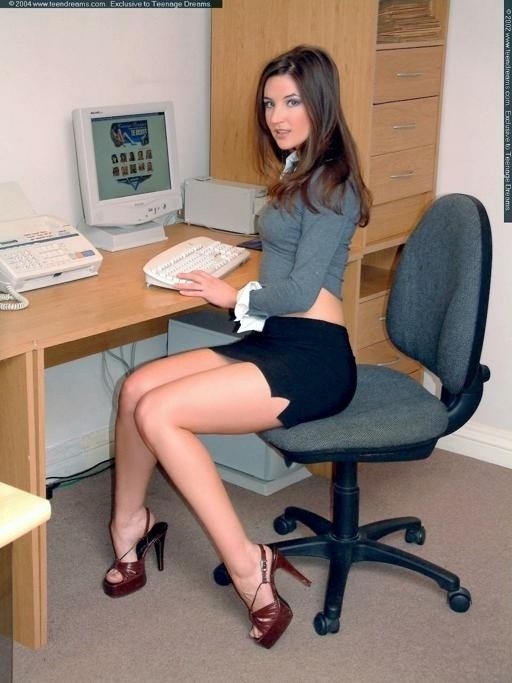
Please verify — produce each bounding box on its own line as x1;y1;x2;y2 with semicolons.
71;100;183;252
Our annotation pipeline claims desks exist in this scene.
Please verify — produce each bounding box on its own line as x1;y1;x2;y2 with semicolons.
0;482;51;683
1;222;364;649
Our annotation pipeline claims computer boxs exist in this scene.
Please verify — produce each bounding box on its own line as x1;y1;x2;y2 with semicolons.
167;310;306;481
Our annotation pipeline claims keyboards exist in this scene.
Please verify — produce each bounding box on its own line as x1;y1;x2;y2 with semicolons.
142;235;251;290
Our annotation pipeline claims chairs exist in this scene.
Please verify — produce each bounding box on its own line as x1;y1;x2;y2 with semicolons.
214;192;493;636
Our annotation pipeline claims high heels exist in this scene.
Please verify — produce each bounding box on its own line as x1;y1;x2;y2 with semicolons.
244;546;313;650
102;501;168;599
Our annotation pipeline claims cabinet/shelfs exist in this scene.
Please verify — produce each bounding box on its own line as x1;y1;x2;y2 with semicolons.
209;1;451;483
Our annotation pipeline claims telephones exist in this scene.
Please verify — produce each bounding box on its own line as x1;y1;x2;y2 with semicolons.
0;214;104;310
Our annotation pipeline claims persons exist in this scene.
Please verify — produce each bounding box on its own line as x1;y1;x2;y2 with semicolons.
112;150;153;176
111;124;137;146
105;43;374;650
